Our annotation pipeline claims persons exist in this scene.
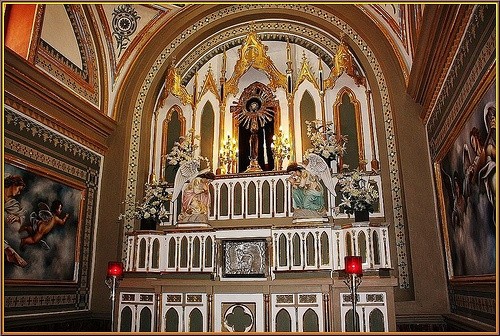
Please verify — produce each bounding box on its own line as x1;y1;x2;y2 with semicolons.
286;164;326;215
180;170;215;215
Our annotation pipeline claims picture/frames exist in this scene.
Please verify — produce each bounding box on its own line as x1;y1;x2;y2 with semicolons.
4;152;88;286
435;62;500;284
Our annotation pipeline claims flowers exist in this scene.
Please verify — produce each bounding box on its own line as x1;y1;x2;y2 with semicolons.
160;128;210;170
331;164;379;216
118;180;173;224
303;120;349;164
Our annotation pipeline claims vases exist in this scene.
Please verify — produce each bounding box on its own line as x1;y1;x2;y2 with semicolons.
140;218;157;230
354;211;370;222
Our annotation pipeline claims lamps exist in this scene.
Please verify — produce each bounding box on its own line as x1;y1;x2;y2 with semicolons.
105;261;123;331
344;256;363;331
